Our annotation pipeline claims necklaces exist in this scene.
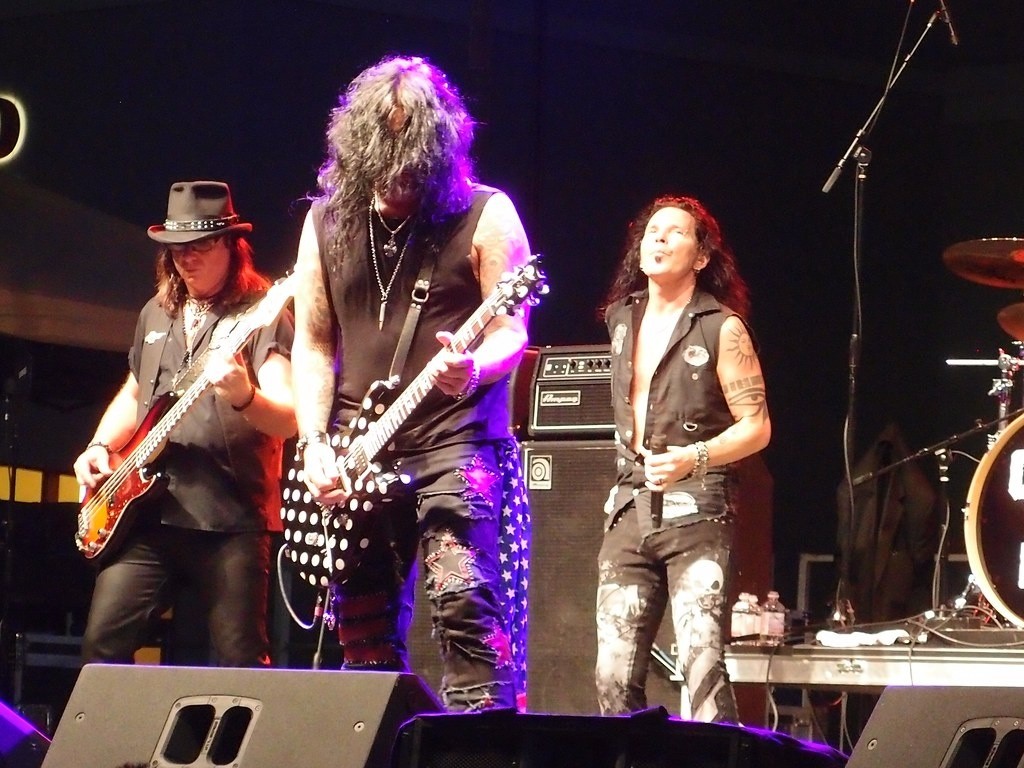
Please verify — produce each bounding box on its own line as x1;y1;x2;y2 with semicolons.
187;293;215;331
368;181;413;332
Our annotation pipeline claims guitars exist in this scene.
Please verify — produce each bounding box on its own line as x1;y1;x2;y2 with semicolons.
281;253;550;591
74;264;296;562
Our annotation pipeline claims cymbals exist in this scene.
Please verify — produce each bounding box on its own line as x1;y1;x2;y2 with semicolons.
943;237;1024;291
997;302;1024;342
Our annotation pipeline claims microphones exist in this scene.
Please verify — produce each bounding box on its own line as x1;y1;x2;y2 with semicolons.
939;0;961;48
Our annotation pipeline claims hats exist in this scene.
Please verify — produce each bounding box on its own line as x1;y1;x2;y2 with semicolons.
147;181;252;245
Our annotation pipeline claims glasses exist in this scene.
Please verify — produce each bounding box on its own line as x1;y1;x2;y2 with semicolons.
164;233;228;255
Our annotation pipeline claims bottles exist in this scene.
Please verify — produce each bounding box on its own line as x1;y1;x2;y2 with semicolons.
730;592;760;653
759;592;786;655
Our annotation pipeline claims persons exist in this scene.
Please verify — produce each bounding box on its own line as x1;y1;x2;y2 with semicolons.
595;195;770;729
291;56;533;712
73;181;296;668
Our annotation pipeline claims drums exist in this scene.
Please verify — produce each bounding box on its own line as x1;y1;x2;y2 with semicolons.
962;412;1024;631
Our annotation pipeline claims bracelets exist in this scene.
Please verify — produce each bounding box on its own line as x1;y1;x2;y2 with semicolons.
452;353;480;401
232;384;255;412
687;441;708;481
295;430;331;463
86;443;113;456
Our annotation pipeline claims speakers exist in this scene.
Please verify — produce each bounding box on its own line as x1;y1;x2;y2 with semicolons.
404;439;773;728
40;664;445;768
844;686;1024;768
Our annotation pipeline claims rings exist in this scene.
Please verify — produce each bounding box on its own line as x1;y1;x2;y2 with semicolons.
657;475;663;485
